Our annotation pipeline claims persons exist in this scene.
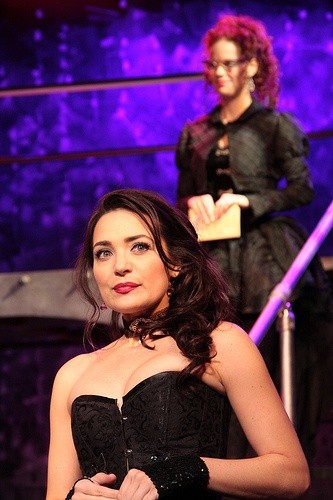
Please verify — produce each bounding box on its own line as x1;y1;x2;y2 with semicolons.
32;188;312;500
173;16;314;383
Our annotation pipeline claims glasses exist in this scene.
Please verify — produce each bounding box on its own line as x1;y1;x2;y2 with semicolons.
202;55;252;69
